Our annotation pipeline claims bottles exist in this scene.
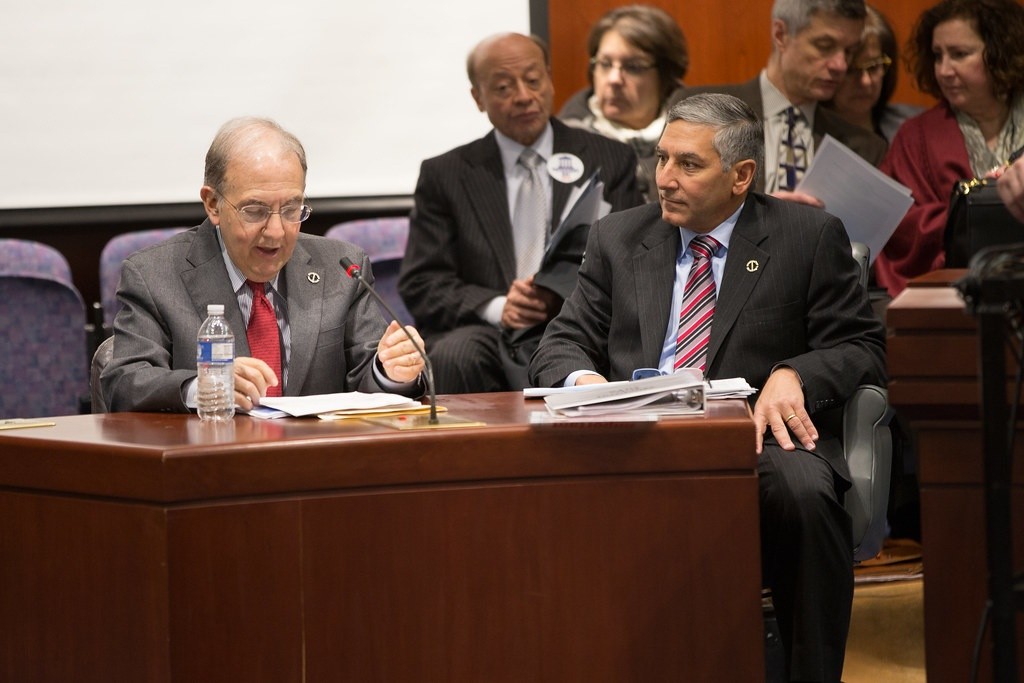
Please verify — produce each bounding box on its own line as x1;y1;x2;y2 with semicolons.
198;305;235;422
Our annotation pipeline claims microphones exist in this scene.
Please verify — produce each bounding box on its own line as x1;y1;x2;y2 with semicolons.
340;257;439;424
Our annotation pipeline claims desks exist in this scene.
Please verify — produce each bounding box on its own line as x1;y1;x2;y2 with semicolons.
0;390;763;683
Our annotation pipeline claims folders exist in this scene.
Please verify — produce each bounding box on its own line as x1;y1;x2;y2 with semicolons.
544;372;706;417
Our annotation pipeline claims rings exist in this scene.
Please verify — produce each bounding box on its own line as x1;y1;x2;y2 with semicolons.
412;353;416;365
785;415;796;423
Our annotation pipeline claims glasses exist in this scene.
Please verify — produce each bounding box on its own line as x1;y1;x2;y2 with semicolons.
848;53;892;79
589;55;659;76
215;188;311;225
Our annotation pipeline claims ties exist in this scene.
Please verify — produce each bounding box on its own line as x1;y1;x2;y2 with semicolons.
513;148;547;279
245;279;282;397
674;234;723;374
778;106;808;192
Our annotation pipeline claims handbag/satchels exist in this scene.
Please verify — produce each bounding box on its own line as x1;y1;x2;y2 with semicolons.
944;146;1024;268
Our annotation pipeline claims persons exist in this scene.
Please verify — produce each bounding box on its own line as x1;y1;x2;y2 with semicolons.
869;0;1023;300
397;33;649;396
649;0;888;208
527;94;888;683
98;120;427;413
817;4;934;147
554;6;690;203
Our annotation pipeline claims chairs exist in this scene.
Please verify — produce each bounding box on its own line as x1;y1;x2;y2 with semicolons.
100;227;192;339
325;218;418;335
0;238;89;421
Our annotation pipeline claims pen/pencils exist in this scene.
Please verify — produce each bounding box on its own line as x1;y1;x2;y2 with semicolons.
0;422;56;430
